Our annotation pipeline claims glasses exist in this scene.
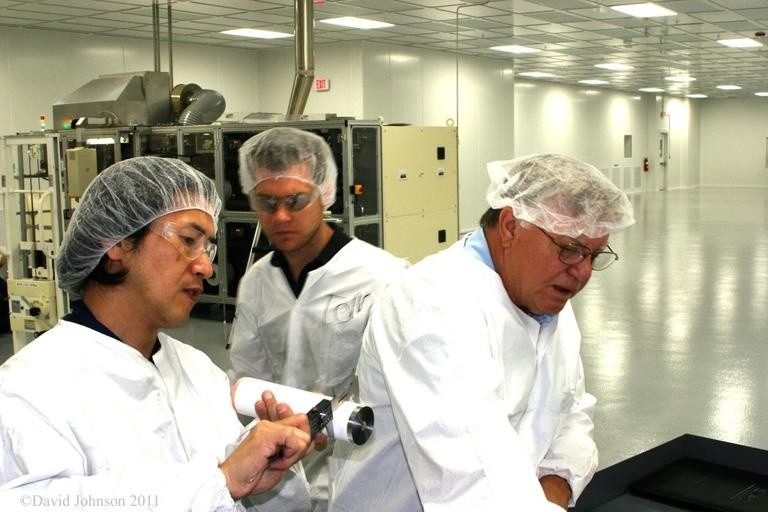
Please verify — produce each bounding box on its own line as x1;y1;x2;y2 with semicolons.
522;217;619;271
145;222;217;265
249;189;320;214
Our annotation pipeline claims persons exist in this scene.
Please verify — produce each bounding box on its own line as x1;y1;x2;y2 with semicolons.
323;153;636;512
0;156;330;512
222;126;410;512
